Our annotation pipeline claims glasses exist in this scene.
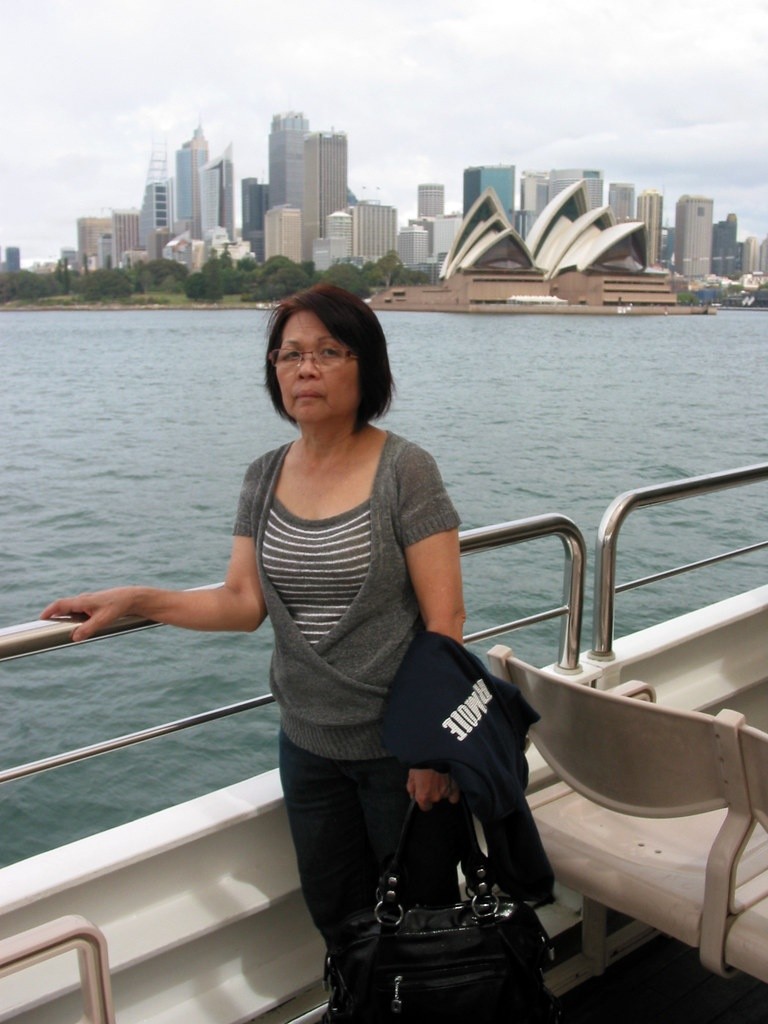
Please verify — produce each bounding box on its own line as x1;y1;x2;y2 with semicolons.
269;348;357;369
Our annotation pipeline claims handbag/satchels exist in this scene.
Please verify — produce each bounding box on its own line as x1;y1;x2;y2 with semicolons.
324;799;555;1024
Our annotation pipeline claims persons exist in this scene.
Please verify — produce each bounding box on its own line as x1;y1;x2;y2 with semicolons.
41;286;465;955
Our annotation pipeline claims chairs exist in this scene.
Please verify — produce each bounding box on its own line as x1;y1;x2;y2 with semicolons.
490;643;768;984
